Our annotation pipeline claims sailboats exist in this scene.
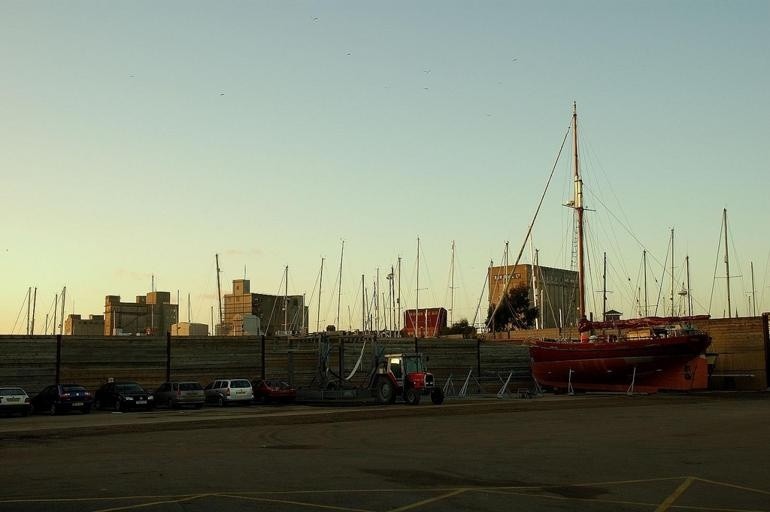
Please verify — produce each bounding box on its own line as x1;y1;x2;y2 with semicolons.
528;100;712;395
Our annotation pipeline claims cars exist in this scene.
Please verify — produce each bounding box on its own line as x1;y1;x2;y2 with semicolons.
0;379;296;417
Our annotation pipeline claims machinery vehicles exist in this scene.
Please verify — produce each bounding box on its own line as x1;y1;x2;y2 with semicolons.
377;353;444;405
